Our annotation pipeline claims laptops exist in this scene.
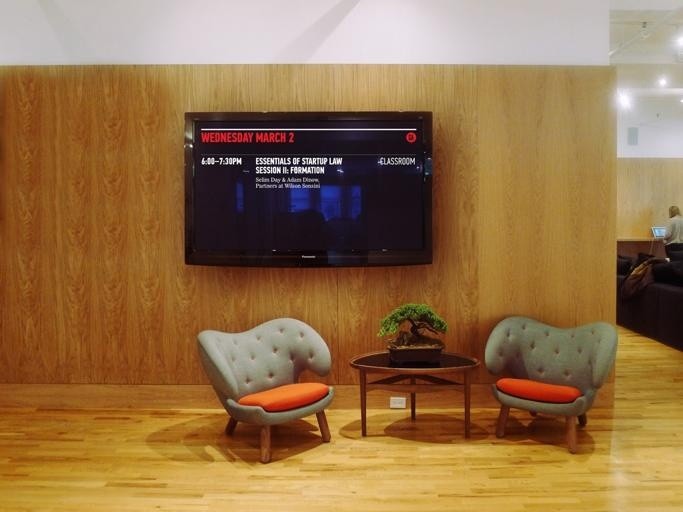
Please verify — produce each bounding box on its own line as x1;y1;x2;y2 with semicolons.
651;226;666;238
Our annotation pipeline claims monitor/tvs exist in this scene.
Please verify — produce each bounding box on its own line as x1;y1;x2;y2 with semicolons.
183;111;432;269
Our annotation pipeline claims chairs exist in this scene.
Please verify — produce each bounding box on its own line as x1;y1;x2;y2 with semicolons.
193;317;338;463
484;317;617;456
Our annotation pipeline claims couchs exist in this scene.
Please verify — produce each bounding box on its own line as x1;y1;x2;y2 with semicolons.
617;251;683;353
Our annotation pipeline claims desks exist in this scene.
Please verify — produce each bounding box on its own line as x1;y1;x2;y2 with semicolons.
348;350;479;440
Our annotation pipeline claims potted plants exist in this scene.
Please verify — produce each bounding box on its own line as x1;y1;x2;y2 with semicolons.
377;303;450;362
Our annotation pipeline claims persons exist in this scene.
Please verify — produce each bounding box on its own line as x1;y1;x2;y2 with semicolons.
663;205;682;263
